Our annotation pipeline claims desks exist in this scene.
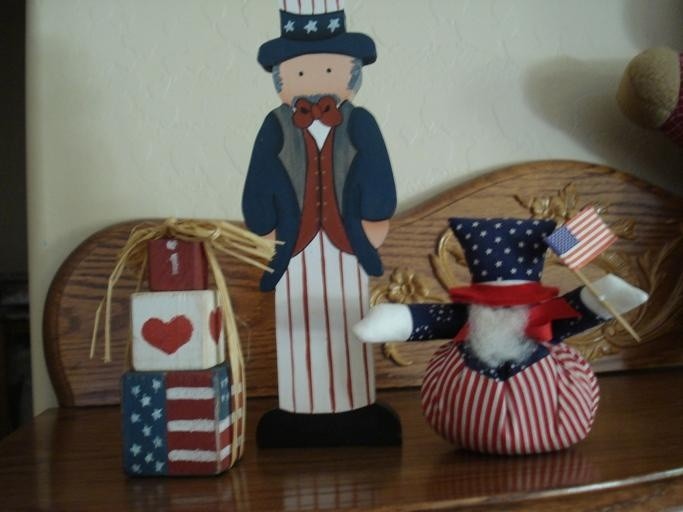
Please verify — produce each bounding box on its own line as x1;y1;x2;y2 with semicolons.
0;157;680;510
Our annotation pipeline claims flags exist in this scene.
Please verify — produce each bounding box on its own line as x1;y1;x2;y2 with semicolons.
545;207;618;271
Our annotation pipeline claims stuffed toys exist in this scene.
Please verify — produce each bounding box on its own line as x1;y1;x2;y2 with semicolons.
617;48;683;145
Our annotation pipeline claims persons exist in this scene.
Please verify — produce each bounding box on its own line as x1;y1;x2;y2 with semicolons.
242;1;402;449
349;215;650;457
